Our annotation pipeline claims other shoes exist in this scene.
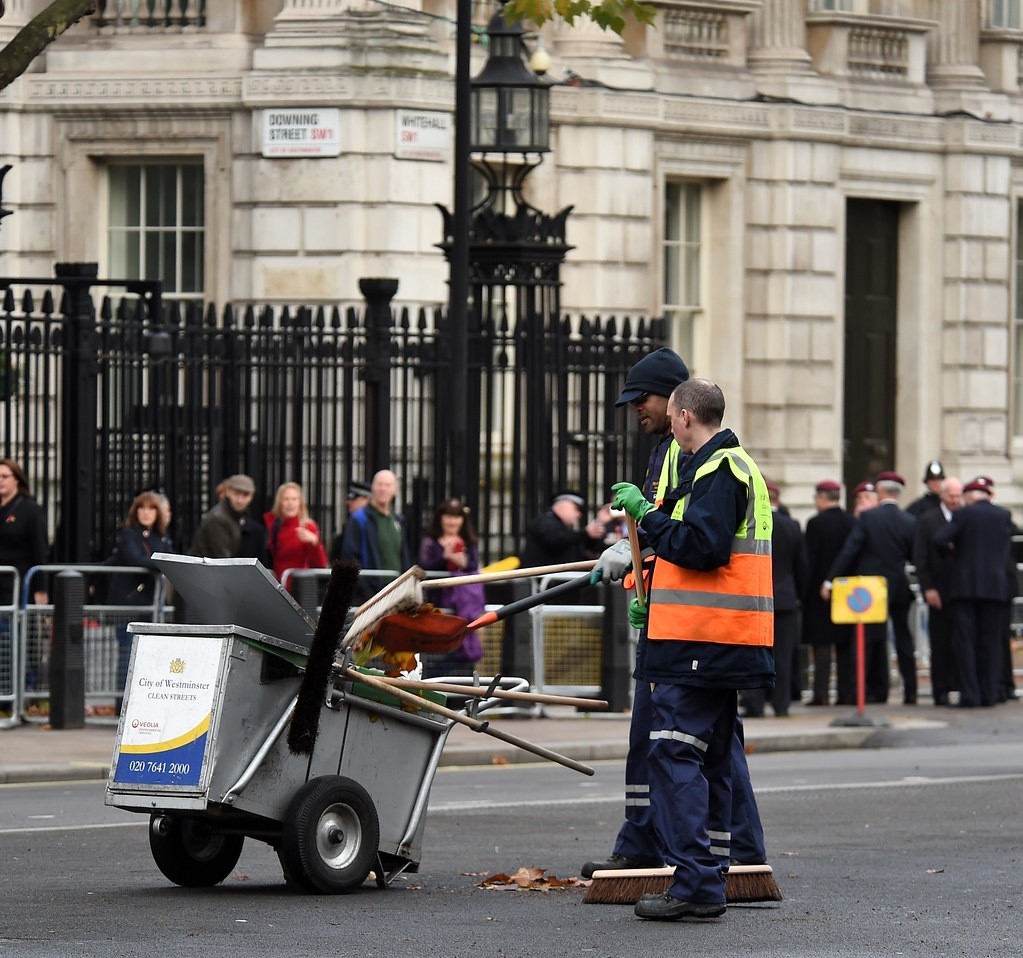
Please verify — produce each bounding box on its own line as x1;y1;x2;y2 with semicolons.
775;708;788;719
739;709;764;717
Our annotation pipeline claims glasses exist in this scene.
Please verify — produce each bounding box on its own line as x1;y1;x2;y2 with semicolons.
627;392;648;408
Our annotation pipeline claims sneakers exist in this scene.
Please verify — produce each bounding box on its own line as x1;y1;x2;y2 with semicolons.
634;892;727;920
580;853;668;879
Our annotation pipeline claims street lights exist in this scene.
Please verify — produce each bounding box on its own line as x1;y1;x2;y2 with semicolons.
434;1;579;562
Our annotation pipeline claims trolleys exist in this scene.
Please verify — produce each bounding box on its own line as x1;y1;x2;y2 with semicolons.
105;553;530;895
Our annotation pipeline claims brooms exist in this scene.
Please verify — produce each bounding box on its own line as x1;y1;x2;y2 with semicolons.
287;557;609;758
343;559;600;649
580;509;784;910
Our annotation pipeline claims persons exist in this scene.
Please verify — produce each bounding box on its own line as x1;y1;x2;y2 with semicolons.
112;490;172;718
332;469;411;609
0;458;51;712
416;498;485;681
611;378;777;919
580;346;767;880
188;473;328;594
524;491;646;607
735;462;1023;719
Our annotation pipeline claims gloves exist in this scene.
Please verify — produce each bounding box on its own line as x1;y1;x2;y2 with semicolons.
611;482;655;525
590;539;633;586
628;598;648;629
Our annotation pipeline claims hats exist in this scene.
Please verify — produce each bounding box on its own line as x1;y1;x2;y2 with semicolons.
767;482;779;492
614;390;643;409
978;477;993;487
621;347;689;397
923;460;945;484
346;480;373;500
853;482;875;495
226;475;255;493
816;481;840;492
962;481;991;496
552;491;587;515
877;472;904;486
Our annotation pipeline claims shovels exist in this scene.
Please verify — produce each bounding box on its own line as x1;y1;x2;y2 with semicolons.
368;566;608;652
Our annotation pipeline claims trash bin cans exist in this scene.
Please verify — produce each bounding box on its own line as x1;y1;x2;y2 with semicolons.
102;550;529;893
